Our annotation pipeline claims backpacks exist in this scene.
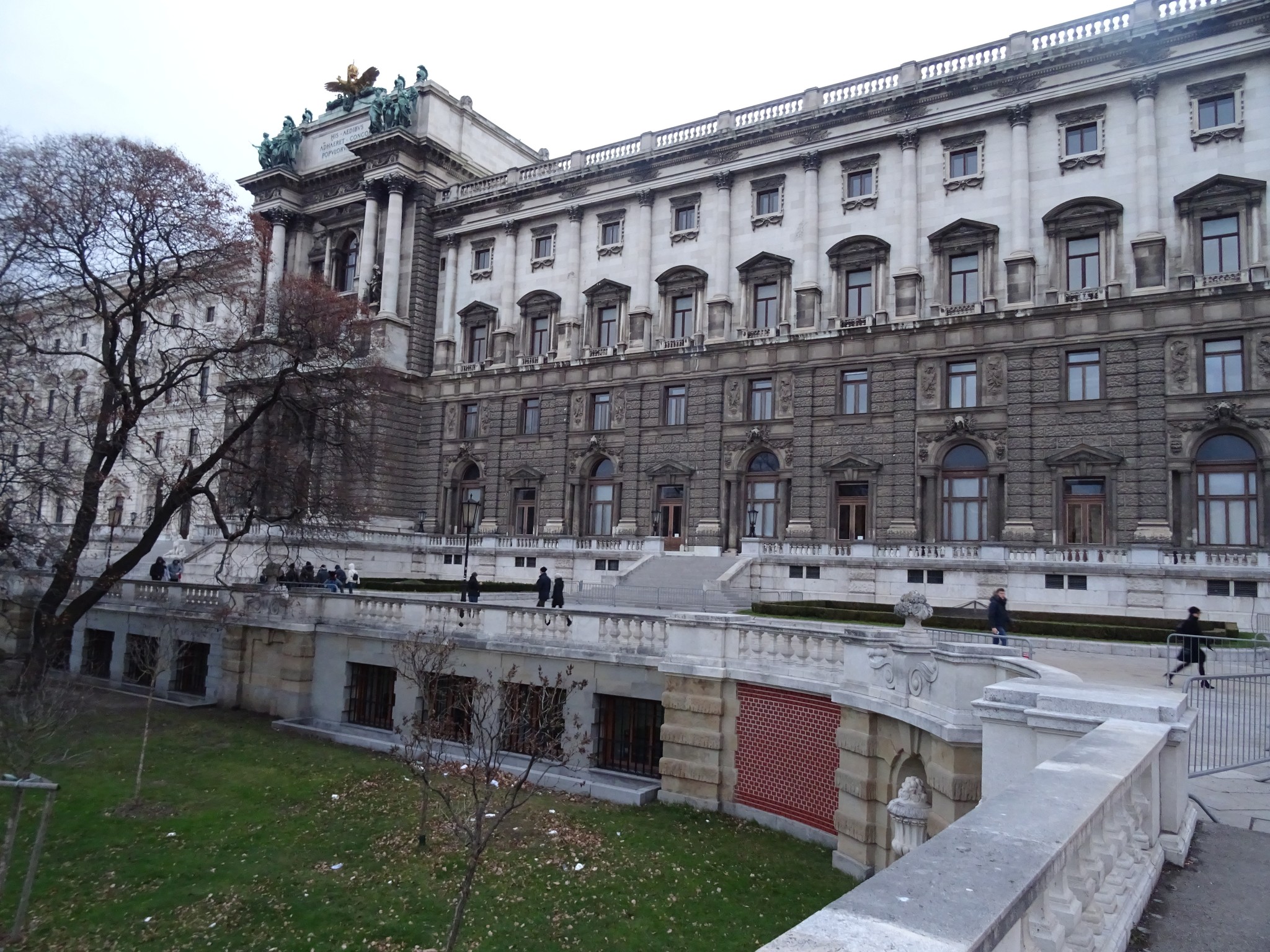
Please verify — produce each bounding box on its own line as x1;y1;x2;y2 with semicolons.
351;570;358;581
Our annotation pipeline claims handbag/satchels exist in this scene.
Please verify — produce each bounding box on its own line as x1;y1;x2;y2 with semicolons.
167;573;171;579
1177;648;1195;663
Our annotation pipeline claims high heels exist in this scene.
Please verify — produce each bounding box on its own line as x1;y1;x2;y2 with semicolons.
1163;671;1175;684
1200;680;1215;689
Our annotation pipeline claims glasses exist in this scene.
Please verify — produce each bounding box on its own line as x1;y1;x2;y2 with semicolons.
1194;612;1201;614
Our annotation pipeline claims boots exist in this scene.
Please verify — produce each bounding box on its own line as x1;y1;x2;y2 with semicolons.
546;617;551;625
567;618;572;626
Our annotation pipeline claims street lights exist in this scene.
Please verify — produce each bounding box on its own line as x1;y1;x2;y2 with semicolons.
458;493;481;627
103;502;125;570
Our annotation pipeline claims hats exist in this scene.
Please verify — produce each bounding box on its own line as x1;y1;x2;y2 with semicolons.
306;561;312;565
289;562;295;568
554;573;562;579
540;566;547;573
472;571;478;576
1188;606;1200;614
328;570;336;577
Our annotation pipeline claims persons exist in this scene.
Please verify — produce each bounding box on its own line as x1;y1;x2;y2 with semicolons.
252;73;413;171
365;264;382;302
167;557;183;582
467;572;481;619
259;561;360;594
1;552;58;574
160;559;171;581
1162;605;1217;689
988;588;1011;645
533;567;551;623
149;556;165;599
546;573;572;628
